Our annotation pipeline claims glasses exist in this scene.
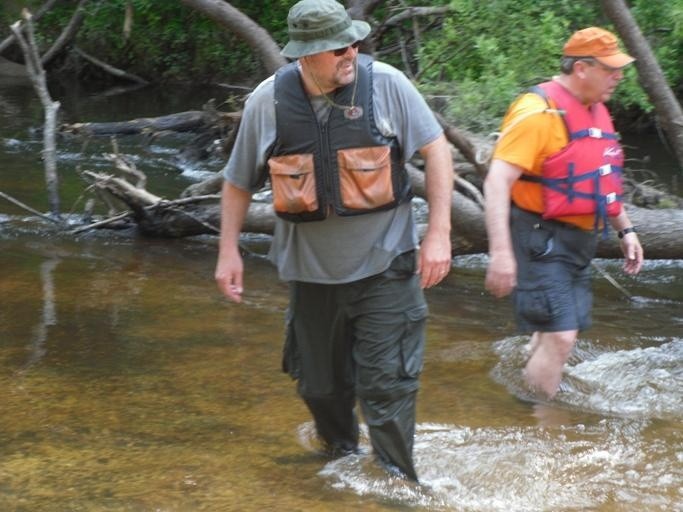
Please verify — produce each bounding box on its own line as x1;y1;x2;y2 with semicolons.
334;41;362;57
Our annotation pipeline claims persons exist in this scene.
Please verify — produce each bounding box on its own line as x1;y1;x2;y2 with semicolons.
480;23;646;398
212;0;456;482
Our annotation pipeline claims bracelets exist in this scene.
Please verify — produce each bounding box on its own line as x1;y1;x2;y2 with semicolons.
616;224;638;239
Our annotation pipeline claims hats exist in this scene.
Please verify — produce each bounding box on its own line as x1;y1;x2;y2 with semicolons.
280;0;372;59
563;27;638;70
